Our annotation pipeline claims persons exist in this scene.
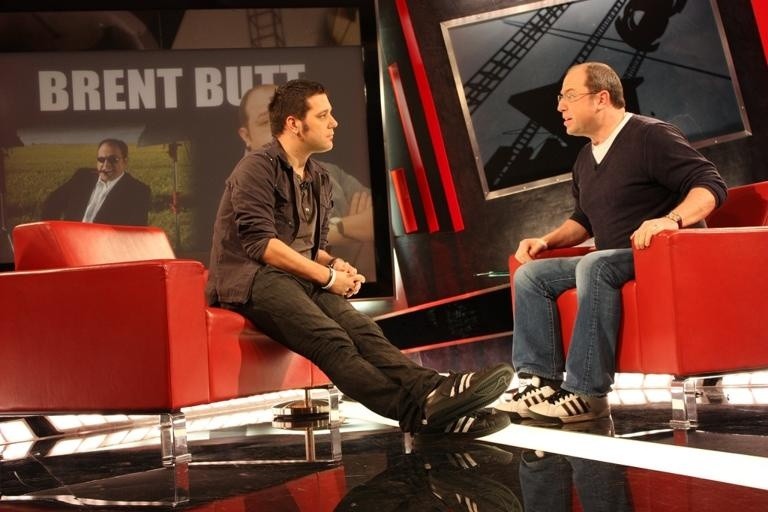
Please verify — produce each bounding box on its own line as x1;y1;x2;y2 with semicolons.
40;139;150;227
495;62;727;423
239;84;377;284
205;80;513;447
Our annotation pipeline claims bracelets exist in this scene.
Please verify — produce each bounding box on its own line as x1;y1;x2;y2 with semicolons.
321;265;337;290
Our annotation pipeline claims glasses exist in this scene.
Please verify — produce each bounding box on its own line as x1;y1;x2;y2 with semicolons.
97;155;123;162
558;92;597;102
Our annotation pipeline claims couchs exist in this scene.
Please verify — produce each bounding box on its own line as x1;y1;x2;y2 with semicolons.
507;184;764;431
0;223;345;469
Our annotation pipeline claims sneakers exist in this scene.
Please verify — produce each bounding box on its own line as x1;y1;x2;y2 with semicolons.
424;364;513;424
411;443;513;468
493;376;557;417
527;388;612;422
427;473;522;511
415;413;510;438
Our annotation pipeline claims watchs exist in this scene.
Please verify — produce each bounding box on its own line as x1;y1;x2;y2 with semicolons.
665;212;683;229
327;257;339;269
